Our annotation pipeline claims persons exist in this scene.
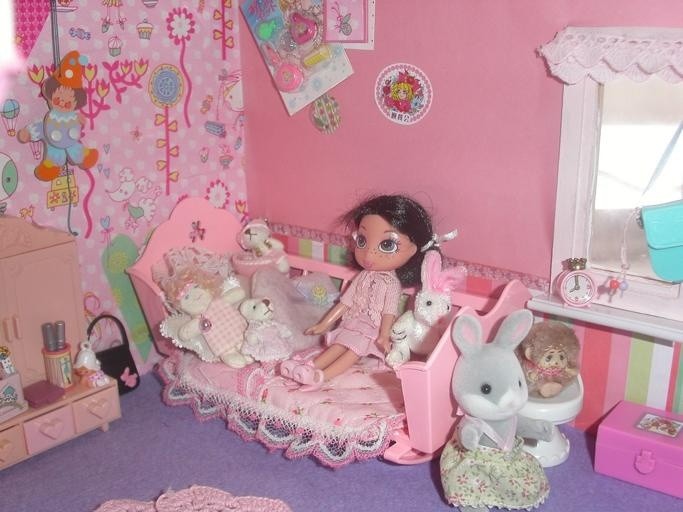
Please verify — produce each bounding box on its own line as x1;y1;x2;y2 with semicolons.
278;195;458;384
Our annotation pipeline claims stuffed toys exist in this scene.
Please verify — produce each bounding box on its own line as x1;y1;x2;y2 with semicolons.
166;272;252;368
520;321;580;398
384;251;467;368
239;297;292;370
235;216;289;277
438;309;556;512
